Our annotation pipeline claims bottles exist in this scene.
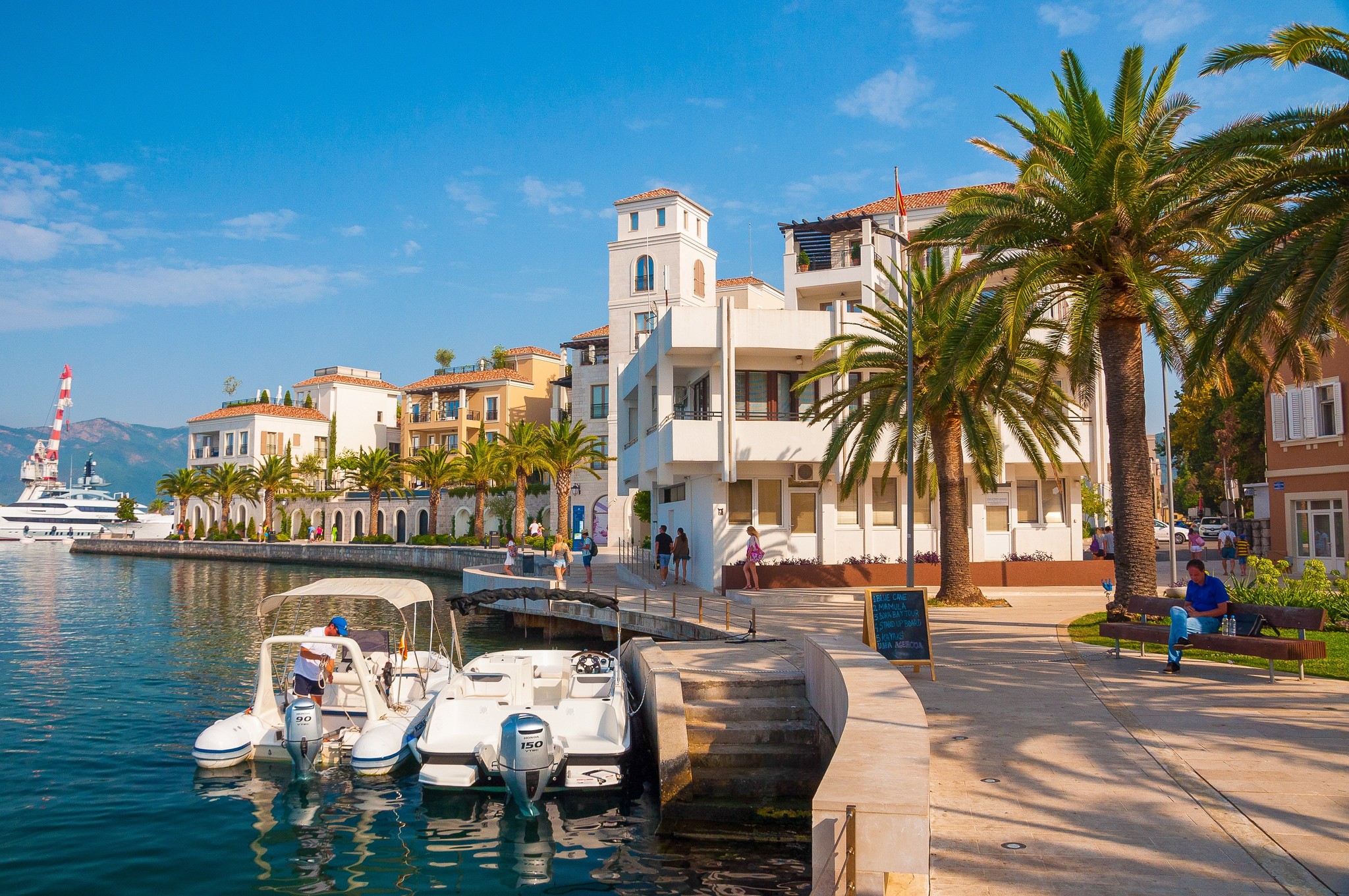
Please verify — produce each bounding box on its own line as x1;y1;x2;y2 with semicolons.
1229;615;1236;636
1222;615;1228;636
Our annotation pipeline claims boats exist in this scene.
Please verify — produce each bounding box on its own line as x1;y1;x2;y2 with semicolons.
413;646;633;802
190;575;456;780
0;357;176;544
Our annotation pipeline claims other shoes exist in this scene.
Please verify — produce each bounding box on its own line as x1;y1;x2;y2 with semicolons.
674;577;678;583
661;581;666;586
682;580;686;585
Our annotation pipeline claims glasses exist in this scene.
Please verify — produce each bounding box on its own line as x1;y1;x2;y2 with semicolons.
333;625;339;634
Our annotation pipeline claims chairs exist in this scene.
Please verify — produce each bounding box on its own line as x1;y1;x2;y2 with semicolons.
463;673;513;698
568;673;614;697
326;625;397;708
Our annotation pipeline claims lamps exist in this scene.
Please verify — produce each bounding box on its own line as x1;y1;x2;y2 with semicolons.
795;355;803;365
717;478;723;486
571;483;580;495
840;292;845;297
683;476;690;482
1003;277;1007;281
828;479;833;486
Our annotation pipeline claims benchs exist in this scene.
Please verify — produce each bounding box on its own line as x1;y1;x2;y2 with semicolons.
1100;596;1326;683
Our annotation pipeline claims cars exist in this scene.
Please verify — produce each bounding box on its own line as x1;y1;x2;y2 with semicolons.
1174;516;1222;540
1153;518;1190;545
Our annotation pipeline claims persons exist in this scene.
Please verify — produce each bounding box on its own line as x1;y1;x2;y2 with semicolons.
742;526;760;591
1162;559;1229;674
672;528;690;585
655;525;673;586
1091;526;1115;561
504;520;593;584
178;522;195;542
1314;526;1331;556
291;617;348;706
257;523;269;543
1188;528;1203;561
308;523;339;544
1218;524;1251;576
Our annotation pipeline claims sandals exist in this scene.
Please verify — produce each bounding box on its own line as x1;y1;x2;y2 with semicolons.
742;584;752;589
752;587;760;591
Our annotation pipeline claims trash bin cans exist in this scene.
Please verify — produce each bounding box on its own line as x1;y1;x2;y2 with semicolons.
489;531;500;549
523;552;534;577
268;531;276;543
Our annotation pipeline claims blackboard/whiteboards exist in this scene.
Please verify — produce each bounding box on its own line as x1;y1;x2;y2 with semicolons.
863;587;935;681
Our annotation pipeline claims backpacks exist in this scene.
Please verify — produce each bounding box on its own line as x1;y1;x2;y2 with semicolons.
584;537;598;557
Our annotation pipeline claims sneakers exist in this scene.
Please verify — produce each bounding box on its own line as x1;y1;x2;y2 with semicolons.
1173;637;1194;651
1162;662;1180;673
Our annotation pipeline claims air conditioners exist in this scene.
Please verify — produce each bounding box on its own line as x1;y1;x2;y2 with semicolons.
652;387;684;411
793;462;822;482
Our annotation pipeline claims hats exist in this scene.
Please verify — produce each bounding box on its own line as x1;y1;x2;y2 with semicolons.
581;529;588;534
538;523;542;527
331;617;348;635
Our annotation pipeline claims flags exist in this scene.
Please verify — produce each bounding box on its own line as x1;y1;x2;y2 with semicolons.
398;632;408;662
897;180;911;241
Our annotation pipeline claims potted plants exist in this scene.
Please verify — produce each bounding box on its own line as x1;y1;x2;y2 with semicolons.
583;355;589;365
1166;577;1187;598
798;250;810;272
851;242;861;266
